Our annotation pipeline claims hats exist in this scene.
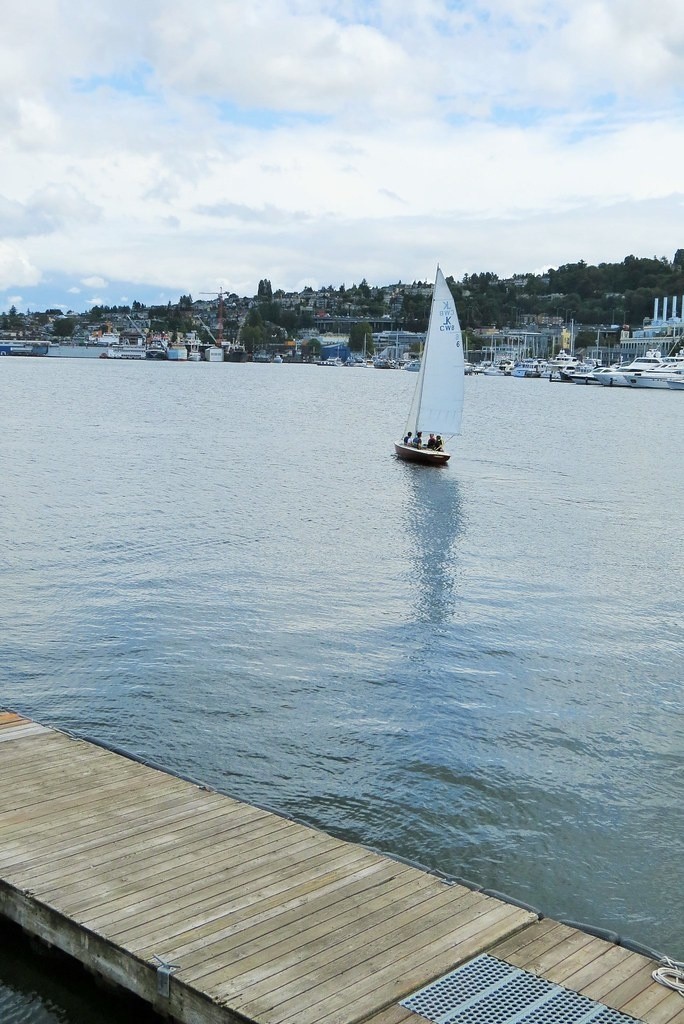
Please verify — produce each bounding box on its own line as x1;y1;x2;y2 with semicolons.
417;432;422;435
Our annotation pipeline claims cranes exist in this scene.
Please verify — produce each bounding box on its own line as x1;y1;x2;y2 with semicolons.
199;287;230;347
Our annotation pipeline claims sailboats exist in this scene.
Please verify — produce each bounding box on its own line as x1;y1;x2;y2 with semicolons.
394;262;464;465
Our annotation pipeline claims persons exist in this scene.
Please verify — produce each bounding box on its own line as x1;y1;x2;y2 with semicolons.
432;435;443;450
427;434;435;448
413;432;422;449
404;432;412;444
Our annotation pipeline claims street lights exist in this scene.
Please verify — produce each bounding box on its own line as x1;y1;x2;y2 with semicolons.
597;329;600;365
508;305;525;326
571;319;574;354
610;307;619;325
620;309;630;326
553;306;576;326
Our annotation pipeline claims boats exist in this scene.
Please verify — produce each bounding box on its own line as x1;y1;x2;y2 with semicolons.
98;335;284;363
372;331;684;390
0;341;51;356
313;351;367;367
